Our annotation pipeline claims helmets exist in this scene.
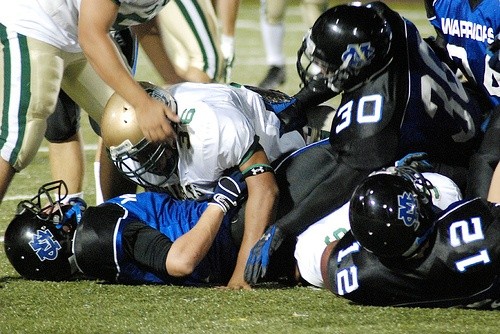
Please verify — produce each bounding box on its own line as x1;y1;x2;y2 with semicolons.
348;172;428;256
4;205;81;281
306;5;390;93
100;81;176;161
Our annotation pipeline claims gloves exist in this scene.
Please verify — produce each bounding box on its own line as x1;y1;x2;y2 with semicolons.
243;225;285;285
210;169;249;215
396;152;428;175
267;98;301;127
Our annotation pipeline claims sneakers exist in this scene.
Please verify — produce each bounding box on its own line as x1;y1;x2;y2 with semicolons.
260;66;285;91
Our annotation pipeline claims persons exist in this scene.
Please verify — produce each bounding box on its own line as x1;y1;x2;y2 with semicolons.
245;0;500;307
0;0;334;290
